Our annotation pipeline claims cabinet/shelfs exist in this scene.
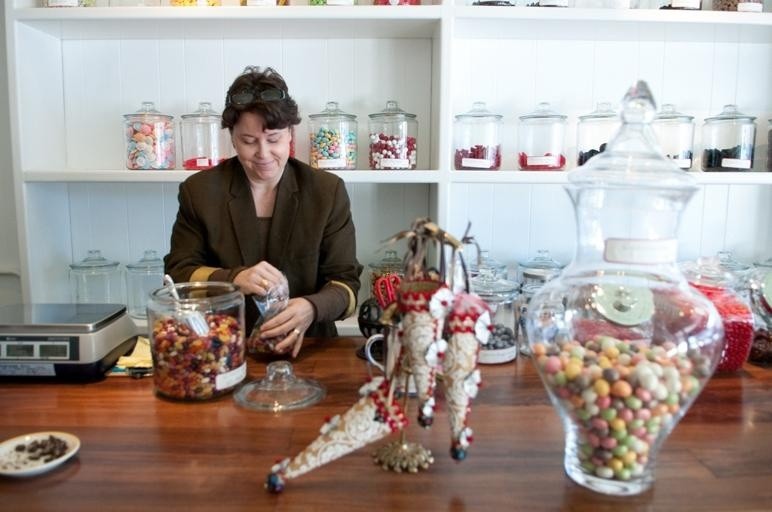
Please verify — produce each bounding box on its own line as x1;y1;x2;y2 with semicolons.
5;2;771;333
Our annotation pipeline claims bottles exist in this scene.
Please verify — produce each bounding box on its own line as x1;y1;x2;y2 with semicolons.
674;249;772;373
368;250;406;304
464;245;564;364
702;102;758;173
650;102;694;171
576;99;622;166
305;101;567;171
528;80;726;494
68;248;249;403
122;100;234;170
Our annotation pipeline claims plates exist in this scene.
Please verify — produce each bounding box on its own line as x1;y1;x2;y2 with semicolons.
0;432;80;482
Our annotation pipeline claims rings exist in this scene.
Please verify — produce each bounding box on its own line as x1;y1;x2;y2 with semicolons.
293;328;301;336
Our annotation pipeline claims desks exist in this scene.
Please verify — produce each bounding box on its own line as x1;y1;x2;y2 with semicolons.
0;328;769;510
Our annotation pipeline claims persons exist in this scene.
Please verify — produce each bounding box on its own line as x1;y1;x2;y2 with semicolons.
163;64;364;359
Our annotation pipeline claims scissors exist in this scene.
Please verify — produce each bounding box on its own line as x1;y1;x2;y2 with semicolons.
375;274;402;310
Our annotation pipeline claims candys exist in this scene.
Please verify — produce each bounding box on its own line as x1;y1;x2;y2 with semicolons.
150;311;248;401
475;317;514;355
684;280;753;375
117;114;755;173
528;334;715;478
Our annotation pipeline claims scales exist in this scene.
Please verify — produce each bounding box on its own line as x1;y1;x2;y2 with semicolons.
0;304;140;385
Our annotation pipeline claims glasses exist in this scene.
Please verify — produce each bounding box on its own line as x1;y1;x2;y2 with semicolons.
227;89;286;105
